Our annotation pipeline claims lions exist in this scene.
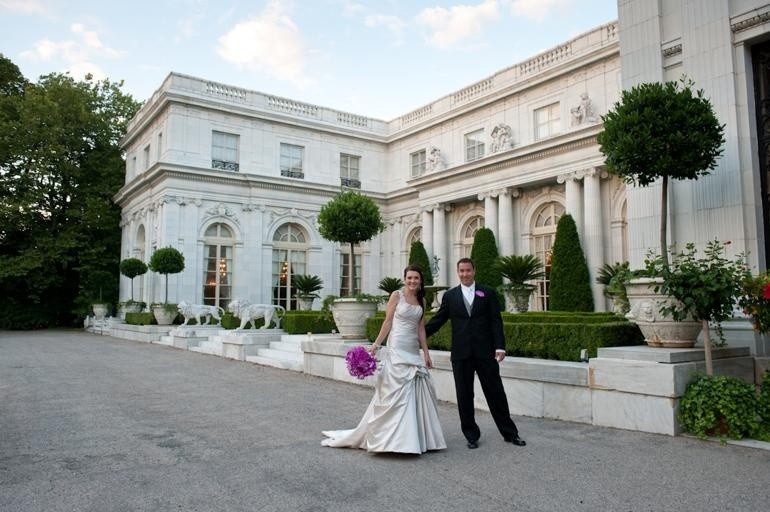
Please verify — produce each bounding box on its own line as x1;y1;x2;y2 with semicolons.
177;300;225;326
228;299;286;330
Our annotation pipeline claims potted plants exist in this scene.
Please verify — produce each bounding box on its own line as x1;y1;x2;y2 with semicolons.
293;274;323;311
498;254;546;314
316;189;387;339
595;261;630;315
376;277;405;311
147;245;185;324
678;372;763;446
85;269;116;318
598;73;726;347
120;258;147;320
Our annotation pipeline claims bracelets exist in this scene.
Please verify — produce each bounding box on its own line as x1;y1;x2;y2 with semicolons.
372;343;382;350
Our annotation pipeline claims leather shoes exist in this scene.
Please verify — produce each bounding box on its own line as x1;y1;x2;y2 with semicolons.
467;440;478;448
504;436;526;446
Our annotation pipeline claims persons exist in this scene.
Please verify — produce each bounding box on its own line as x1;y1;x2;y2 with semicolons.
322;265;446;456
425;258;527;448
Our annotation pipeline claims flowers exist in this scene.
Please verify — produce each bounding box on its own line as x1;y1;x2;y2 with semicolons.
345;346;380;380
